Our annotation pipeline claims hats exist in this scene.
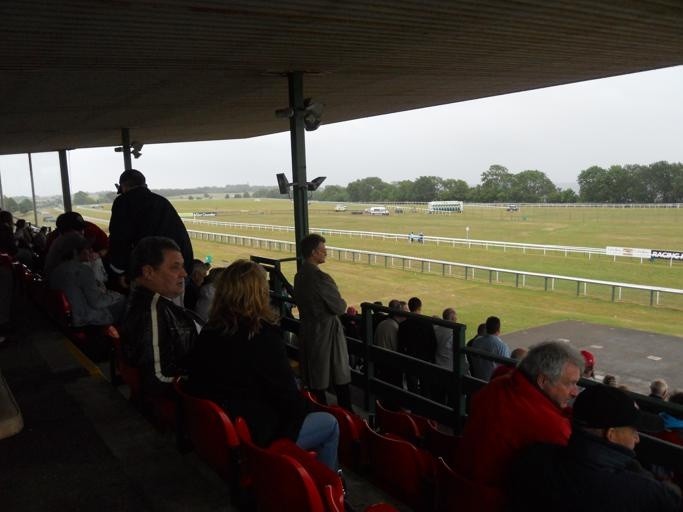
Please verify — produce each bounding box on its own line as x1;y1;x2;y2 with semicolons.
116;170;146;193
581;349;594;368
572;385;664;432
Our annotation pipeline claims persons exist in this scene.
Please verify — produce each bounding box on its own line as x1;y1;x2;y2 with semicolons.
184;260;342;477
2;169;225;390
292;233;353;414
344;297;682;512
410;232;423;243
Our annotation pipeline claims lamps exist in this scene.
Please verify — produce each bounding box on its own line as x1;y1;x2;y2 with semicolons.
273;98;325;131
114;141;144;158
276;172;326;195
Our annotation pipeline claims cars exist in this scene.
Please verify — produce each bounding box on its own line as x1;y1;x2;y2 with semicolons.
334;203;370;215
34;205;56;224
506;205;518;212
90;204;104;209
393;206;404;214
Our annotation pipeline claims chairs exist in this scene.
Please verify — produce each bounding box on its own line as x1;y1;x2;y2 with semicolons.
0;253;495;511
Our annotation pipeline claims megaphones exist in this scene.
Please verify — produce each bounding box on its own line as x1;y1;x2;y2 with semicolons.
304;97;327;120
304;113;321;131
132;150;141;158
131;140;143;150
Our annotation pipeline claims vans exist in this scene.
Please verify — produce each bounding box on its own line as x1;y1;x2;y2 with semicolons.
369;206;389;216
